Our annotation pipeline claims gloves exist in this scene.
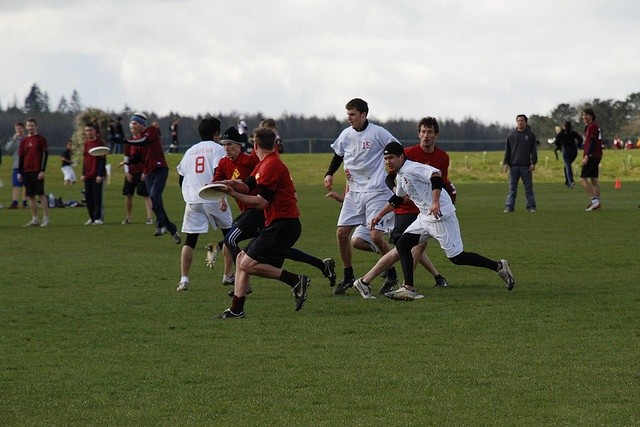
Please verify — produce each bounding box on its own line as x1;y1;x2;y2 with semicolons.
112;137;126;144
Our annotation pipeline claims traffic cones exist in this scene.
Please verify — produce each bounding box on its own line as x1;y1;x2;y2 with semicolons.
613;177;623;189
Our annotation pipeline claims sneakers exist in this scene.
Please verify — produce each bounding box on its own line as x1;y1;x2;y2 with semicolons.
176;282;188;290
222;275;234;285
169;225;181;244
40;220;49;227
384;286;416;301
290;275;310;311
212;309;245;319
529;206;536;212
205;242;219;270
22;204;29;209
503;208;514;212
353;277;376;299
23;221;39;226
7;205;20;209
379;276;398;294
153;228;165;235
586;202;601;211
433;278;447;288
497;259;514;290
146;218;153;225
122;218;131;225
85;218;93;225
333;277;355;294
93;219;103;225
228;286;253;297
322;257;336;286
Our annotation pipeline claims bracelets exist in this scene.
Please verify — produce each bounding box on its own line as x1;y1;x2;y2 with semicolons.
388;194;404;209
429;176;444;190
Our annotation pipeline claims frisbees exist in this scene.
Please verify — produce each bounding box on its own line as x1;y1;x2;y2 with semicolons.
90;147;110;157
199;183;229;201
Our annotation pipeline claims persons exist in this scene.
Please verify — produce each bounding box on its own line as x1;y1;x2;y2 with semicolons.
5;121;29;208
209;126;337;297
553;126;564;160
325;182;449;287
325;185;397;278
168;116;180;153
261;119;285;153
107;119;115;154
60;139;77;188
80;122;106;226
121;120;154;225
384;116;457;289
110;112;181;244
16;117;50;228
580;108;603;212
152;122;160;139
324;98;403;294
113;117;124;153
236;115;248;152
176;117;235;291
555;121;583;189
502;114;538;213
368;142;515;300
211;128;311;319
612;133;640;148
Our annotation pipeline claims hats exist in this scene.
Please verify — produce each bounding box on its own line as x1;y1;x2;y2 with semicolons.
384;141;404;154
221;127;241;144
